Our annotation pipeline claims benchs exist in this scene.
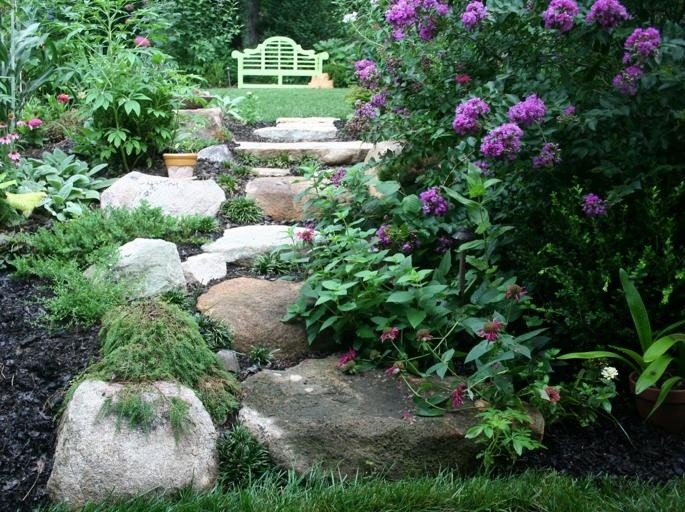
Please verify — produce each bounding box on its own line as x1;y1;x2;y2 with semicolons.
231;36;329;89
